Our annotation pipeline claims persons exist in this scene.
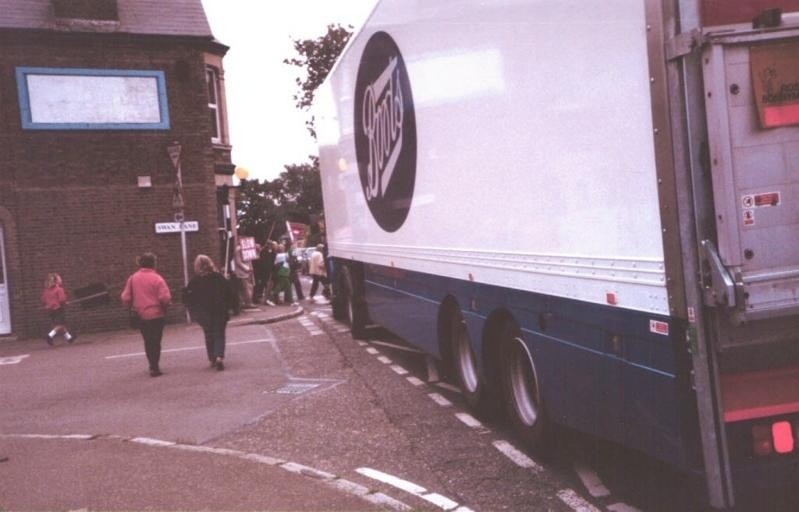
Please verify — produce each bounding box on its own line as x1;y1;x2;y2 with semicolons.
183;254;233;371
40;272;77;346
121;252;172;377
233;235;333;308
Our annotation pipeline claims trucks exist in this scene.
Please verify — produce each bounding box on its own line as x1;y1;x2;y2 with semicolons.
308;2;799;512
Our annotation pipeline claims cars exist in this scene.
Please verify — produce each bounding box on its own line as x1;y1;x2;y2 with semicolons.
271;246;316;275
